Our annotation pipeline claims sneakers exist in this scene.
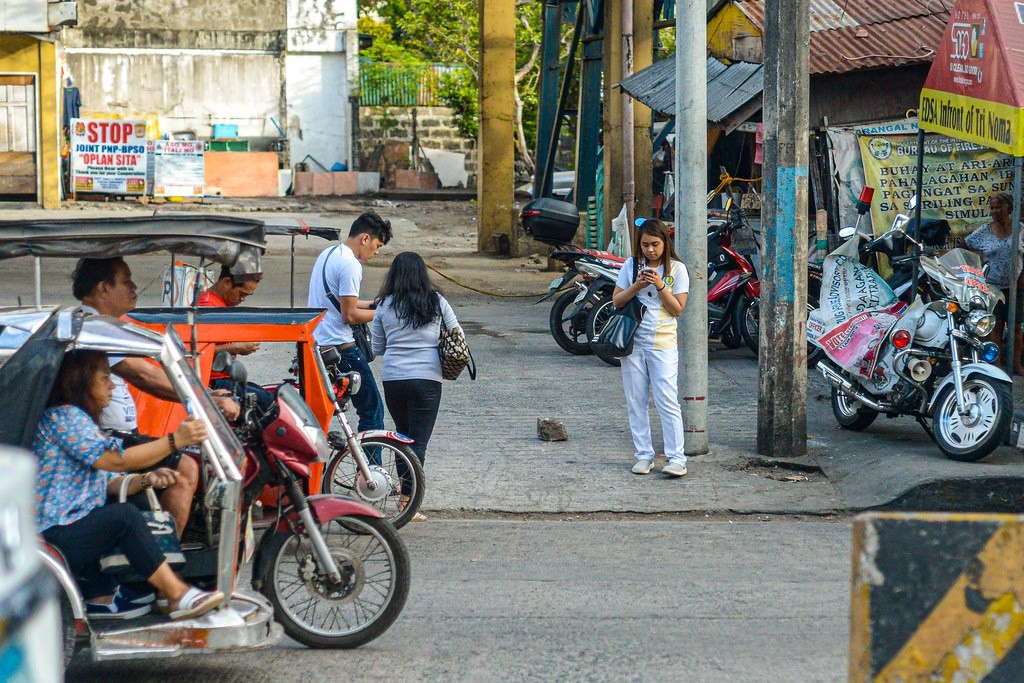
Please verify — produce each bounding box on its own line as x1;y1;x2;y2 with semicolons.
114;586;155;604
662;461;687;476
631;460;655;473
83;596;151;620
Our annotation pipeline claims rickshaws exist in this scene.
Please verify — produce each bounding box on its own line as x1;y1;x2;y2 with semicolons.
0;210;428;536
0;307;411;681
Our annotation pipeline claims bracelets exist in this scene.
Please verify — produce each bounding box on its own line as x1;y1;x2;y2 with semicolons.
167;432;179;452
140;472;147;488
656;284;665;292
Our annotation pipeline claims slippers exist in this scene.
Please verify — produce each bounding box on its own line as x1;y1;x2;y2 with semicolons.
167;588;226;619
155;597;168;606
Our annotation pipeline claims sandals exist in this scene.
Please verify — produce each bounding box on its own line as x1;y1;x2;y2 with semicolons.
389;483;402;496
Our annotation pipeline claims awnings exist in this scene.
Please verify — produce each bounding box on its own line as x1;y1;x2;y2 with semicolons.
611;50;763;136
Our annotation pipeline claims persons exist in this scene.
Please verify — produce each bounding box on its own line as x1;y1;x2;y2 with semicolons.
611;218;690;476
29;349;227;622
956;193;1024;376
371;251;465;522
308;207;398;497
187;262;281;442
70;258;241;544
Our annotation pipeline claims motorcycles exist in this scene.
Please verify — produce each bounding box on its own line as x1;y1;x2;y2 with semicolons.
519;197;829;368
813;187;1013;462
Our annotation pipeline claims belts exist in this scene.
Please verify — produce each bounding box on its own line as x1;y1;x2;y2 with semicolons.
319;341;356;352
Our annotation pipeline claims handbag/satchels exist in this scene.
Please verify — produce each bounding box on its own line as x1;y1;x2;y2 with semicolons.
589;256;647;357
349;321;376;362
437;293;476;381
100;472;189;572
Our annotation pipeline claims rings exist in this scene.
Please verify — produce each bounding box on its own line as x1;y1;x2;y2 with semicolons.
159;485;167;489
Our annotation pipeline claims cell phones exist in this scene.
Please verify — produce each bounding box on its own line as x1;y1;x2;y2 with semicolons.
641;268;654;280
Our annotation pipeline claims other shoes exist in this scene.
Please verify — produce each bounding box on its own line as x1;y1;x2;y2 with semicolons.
396;506;427;523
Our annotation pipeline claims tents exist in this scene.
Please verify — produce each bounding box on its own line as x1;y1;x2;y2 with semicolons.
911;0;1024;395
